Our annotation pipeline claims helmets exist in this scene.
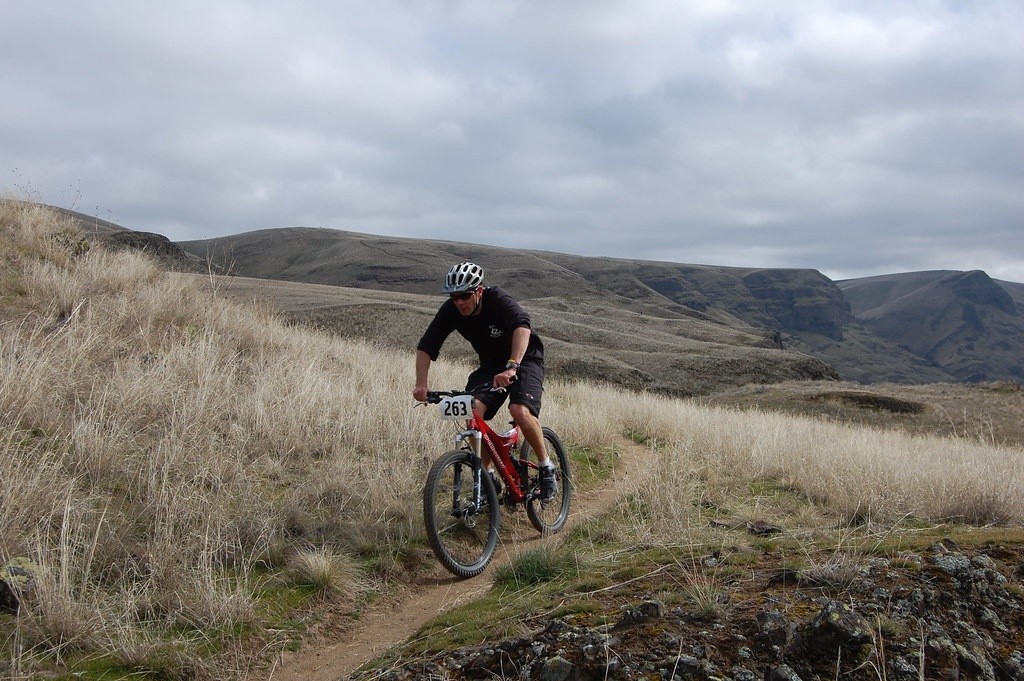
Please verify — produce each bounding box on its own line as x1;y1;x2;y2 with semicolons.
440;262;484;295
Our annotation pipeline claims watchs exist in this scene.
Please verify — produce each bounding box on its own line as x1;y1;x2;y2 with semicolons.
505;362;520;372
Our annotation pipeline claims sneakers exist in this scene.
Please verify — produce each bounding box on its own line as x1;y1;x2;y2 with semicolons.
468;475;502;502
539;464;558;504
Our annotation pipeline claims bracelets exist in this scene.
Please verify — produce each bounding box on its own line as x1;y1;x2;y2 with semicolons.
508;360;515;363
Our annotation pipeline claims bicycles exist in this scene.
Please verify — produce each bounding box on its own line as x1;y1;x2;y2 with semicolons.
411;374;571;580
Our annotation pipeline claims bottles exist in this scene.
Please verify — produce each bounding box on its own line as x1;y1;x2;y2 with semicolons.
509;453;525;479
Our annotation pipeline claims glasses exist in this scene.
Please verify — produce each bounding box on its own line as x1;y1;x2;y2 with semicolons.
450;285;480;300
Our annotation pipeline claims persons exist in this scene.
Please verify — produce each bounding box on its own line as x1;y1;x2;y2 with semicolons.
413;262;557;506
